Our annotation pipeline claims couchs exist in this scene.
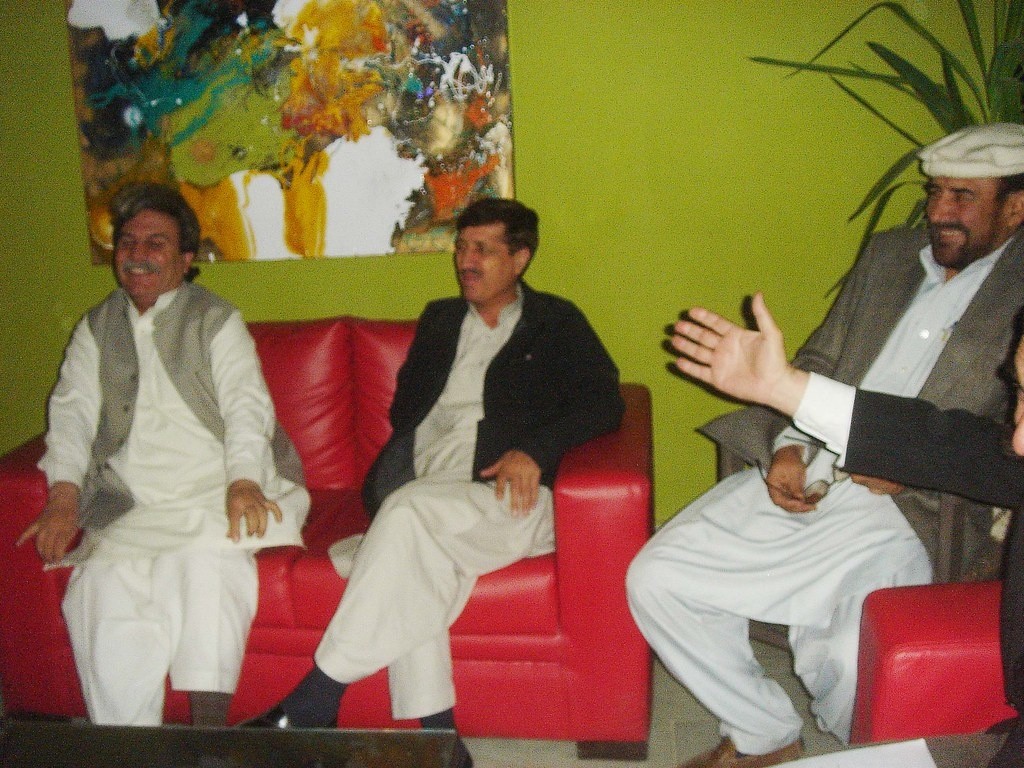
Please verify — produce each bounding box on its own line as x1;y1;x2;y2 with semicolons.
0;313;654;759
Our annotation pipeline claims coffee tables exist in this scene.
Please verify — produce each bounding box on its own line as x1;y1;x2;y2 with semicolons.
0;721;476;768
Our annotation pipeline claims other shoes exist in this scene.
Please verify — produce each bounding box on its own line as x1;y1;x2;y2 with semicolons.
987;713;1024;768
675;735;808;768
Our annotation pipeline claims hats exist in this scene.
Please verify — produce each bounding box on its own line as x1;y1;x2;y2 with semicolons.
917;121;1024;180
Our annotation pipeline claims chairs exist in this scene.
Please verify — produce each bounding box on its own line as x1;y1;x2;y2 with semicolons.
710;418;1010;651
849;581;1020;741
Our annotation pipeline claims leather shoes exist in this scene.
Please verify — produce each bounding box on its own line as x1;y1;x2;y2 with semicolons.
238;702;339;728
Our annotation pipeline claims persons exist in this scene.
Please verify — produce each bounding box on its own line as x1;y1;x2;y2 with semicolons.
16;185;312;726
626;123;1024;768
672;292;1024;768
234;199;624;768
